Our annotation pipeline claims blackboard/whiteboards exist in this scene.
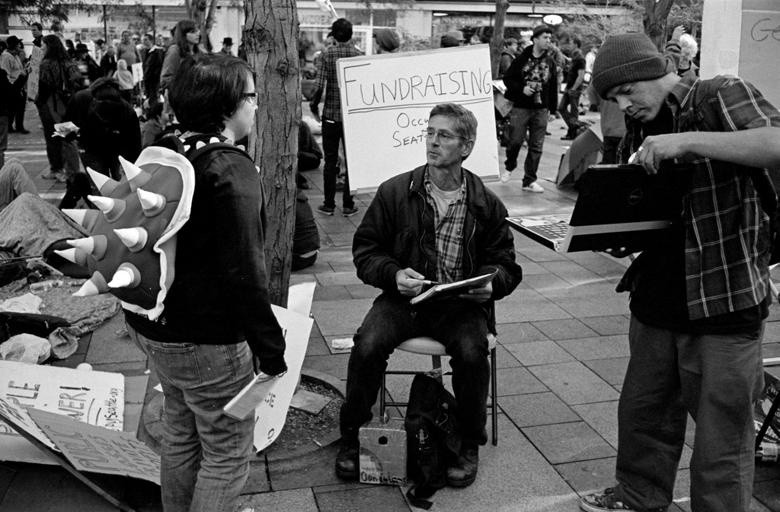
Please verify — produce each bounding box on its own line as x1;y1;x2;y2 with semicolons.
336;44;501;196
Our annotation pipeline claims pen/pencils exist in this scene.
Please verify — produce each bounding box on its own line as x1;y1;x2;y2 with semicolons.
407;278;442;284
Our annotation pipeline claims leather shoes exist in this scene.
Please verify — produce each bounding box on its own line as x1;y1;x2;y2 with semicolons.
448;434;479;488
335;436;360;481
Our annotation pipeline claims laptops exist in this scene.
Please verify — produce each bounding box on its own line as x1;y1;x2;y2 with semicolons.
503;162;683;253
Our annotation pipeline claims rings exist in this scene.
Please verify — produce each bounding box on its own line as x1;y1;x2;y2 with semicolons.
637;145;643;153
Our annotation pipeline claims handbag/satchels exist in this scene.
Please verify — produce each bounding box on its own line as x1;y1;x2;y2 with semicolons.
504;85;523;104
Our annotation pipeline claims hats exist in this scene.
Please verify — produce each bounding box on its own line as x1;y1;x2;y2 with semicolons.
529;24;555;41
591;31;673;101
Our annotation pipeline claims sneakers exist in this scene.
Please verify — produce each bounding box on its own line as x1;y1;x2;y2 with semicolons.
521;181;545;193
341;207;359;217
41;171;70;183
578;485;669;512
316;204;335;216
500;169;512;184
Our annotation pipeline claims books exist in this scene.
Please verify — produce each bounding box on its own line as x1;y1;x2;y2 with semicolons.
224;373;281;422
409;270;499;305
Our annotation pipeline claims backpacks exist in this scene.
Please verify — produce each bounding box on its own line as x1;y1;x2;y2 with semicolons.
53;138;255;325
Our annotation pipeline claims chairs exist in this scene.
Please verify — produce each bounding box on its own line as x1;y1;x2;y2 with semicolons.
374;302;501;447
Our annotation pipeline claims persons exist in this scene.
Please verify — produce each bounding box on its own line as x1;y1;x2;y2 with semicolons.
1;20;234;183
337;102;523;489
126;55;286;512
678;35;698;76
291;19;399;271
442;24;627;193
580;32;779;511
